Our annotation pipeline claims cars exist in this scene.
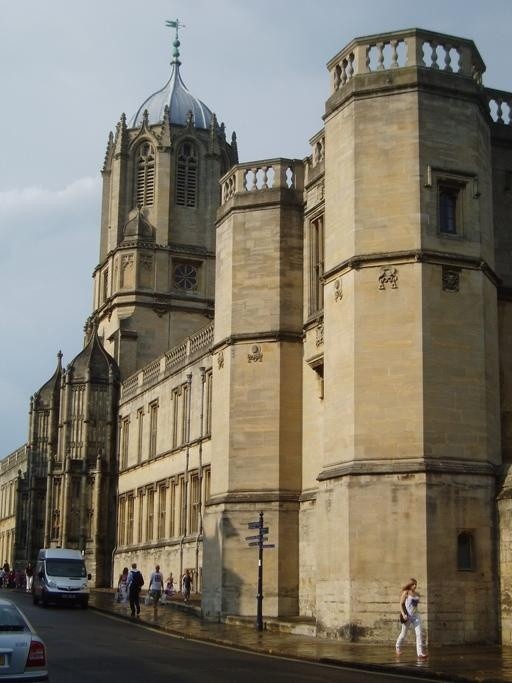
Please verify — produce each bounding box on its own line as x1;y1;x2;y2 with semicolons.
1;598;48;682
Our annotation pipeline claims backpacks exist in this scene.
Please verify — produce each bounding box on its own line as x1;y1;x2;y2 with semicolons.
131;571;144;586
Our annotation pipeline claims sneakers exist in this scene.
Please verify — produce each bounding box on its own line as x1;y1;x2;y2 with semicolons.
419;653;427;657
396;648;402;655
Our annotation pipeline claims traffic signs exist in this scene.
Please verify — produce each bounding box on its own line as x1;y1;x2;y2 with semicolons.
243;520;276;549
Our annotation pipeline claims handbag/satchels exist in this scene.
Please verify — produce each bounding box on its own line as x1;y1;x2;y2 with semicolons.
181;588;188;594
401;604;408;623
145;598;152;606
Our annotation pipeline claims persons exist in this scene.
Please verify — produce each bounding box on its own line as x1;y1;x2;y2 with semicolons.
396;578;428;657
0;560;34;593
117;563;193;615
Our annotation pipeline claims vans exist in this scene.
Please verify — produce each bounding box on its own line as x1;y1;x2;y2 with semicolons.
31;546;93;606
